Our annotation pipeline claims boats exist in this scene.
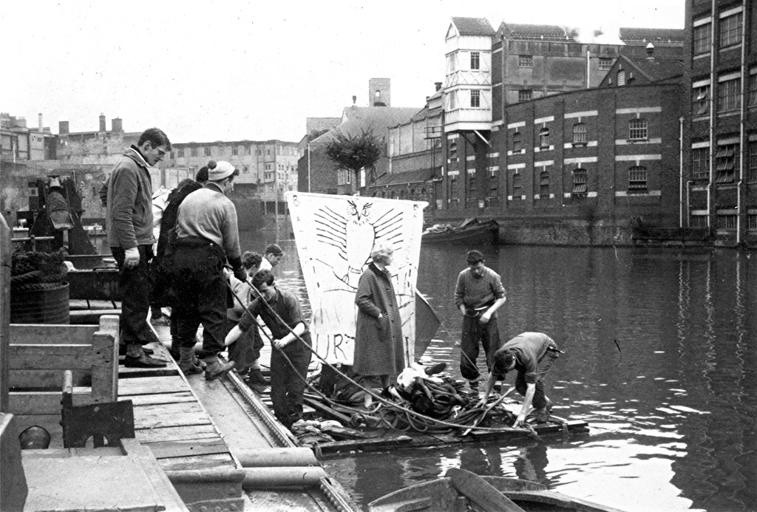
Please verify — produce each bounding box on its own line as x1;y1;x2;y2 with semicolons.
422;216;500;244
368;467;623;512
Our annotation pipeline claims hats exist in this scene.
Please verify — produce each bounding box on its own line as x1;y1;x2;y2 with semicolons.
207;160;236;181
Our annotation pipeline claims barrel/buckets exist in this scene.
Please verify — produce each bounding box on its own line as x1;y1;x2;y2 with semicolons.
10;280;71;326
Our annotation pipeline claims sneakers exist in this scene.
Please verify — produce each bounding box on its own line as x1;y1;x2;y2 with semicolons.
536;407;548;423
530;396;552;417
364;394;373;409
150;313;171;326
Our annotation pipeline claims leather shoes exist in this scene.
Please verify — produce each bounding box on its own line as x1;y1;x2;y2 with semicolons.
205;358;235;380
124;352;167;368
119;343;153;355
192;354;206;371
250;367;271;385
178;357;201;375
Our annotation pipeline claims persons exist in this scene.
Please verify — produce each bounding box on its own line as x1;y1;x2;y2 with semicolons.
220;252;272;384
101;128;171;368
150;179;194;327
178;159;248;381
454;249;508;396
473;330;560;428
354;246;405;410
228;244;283;363
223;269;313;434
156;166;208;361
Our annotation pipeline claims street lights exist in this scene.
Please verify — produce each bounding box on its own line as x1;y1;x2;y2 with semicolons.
677;114;687;228
306;140;312;193
